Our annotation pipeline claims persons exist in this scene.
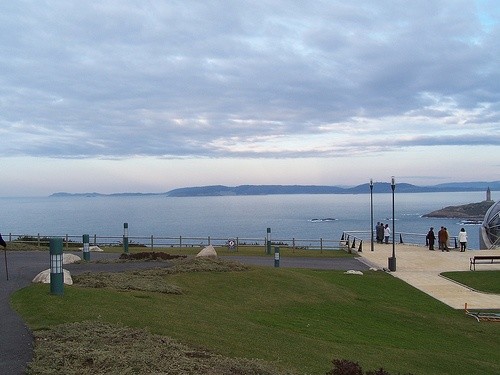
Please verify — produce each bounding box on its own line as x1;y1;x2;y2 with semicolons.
0;233;6;248
458;228;467;252
377;223;384;244
376;222;380;243
384;224;391;244
438;226;449;251
428;227;436;250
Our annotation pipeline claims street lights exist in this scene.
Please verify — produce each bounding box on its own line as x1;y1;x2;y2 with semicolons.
369;177;374;251
390;177;395;257
